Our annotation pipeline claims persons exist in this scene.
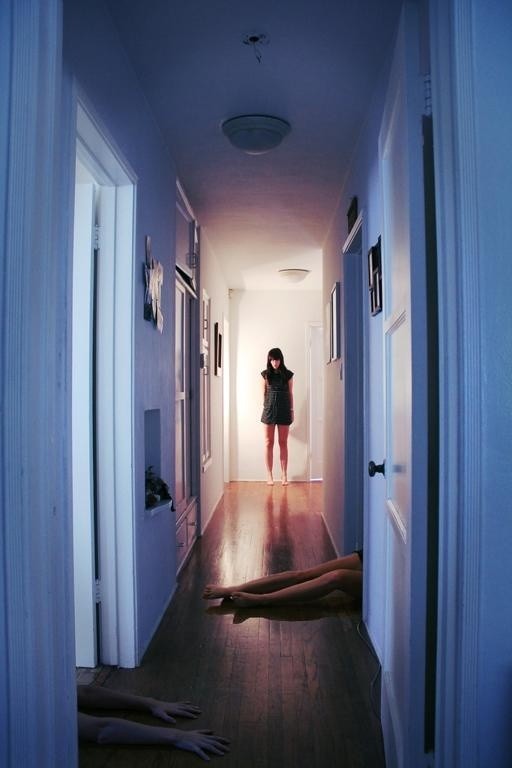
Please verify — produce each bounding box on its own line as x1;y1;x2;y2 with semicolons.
201;549;363;608
77;685;231;761
260;347;295;485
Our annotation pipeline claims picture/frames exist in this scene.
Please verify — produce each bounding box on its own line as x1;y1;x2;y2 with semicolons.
329;283;341;363
214;322;225;377
368;235;382;316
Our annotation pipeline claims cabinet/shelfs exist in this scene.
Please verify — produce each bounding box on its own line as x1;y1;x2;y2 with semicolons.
173;202;200;295
175;494;198;575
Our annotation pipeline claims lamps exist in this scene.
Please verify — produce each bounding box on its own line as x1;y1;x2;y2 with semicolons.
221;114;291;156
278;269;310;284
